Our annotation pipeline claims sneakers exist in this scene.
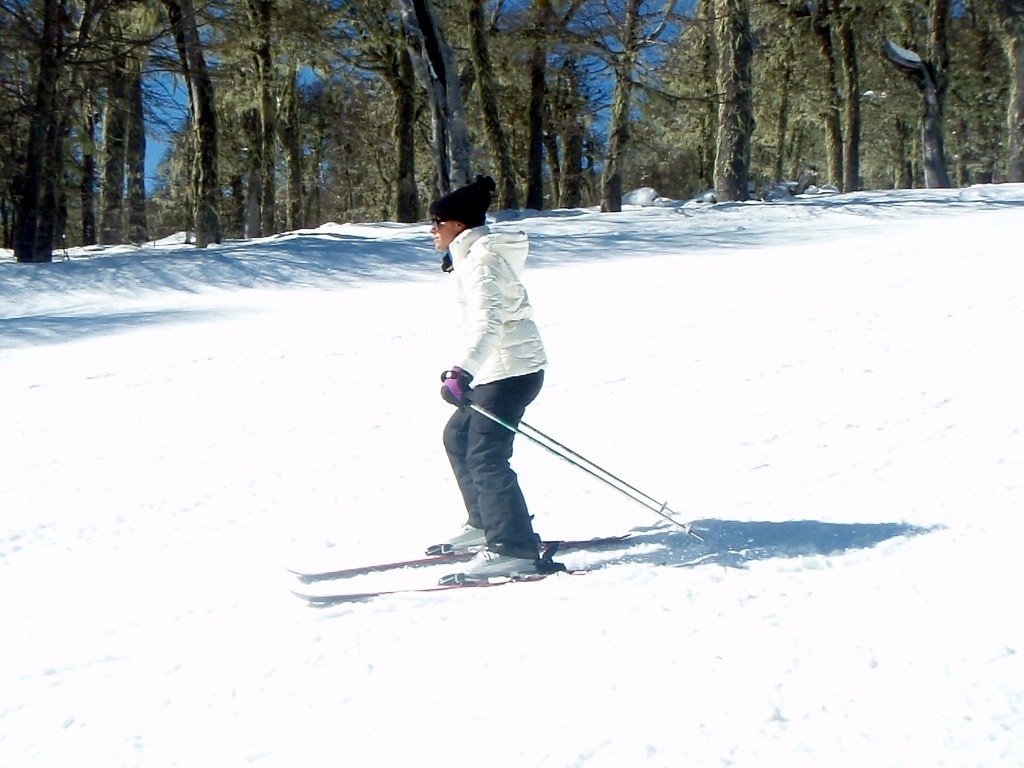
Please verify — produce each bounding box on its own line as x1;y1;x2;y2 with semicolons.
441;550;542;585
425;525;489;555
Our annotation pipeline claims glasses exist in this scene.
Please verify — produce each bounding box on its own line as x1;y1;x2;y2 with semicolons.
428;218;449;232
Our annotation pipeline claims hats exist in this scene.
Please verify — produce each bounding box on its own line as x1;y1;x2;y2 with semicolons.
427;176;500;227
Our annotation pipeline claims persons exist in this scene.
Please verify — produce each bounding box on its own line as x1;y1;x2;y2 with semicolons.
425;176;549;579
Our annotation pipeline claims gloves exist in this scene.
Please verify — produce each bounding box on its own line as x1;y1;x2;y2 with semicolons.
440;366;474;413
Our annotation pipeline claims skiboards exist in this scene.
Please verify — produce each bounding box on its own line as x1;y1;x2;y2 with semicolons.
283;533;666;602
